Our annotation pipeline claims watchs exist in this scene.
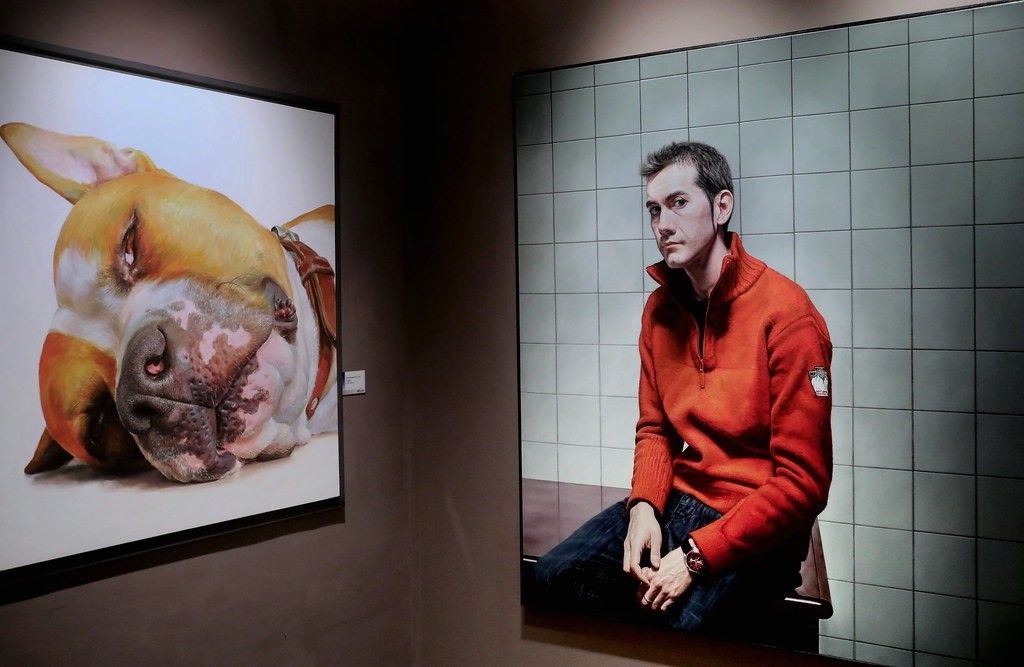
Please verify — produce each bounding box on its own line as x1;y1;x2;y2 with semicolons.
680;539;706;576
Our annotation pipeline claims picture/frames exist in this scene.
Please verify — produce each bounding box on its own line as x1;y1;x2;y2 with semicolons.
0;32;345;589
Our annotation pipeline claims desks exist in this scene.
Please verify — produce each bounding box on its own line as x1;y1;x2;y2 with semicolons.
522;477;833;655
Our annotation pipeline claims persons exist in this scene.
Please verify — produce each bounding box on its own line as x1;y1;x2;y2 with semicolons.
522;142;833;640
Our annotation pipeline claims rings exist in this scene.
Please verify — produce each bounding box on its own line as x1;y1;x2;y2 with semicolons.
643;596;652;603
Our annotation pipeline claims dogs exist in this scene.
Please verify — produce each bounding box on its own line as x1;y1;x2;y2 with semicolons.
0;121;336;482
